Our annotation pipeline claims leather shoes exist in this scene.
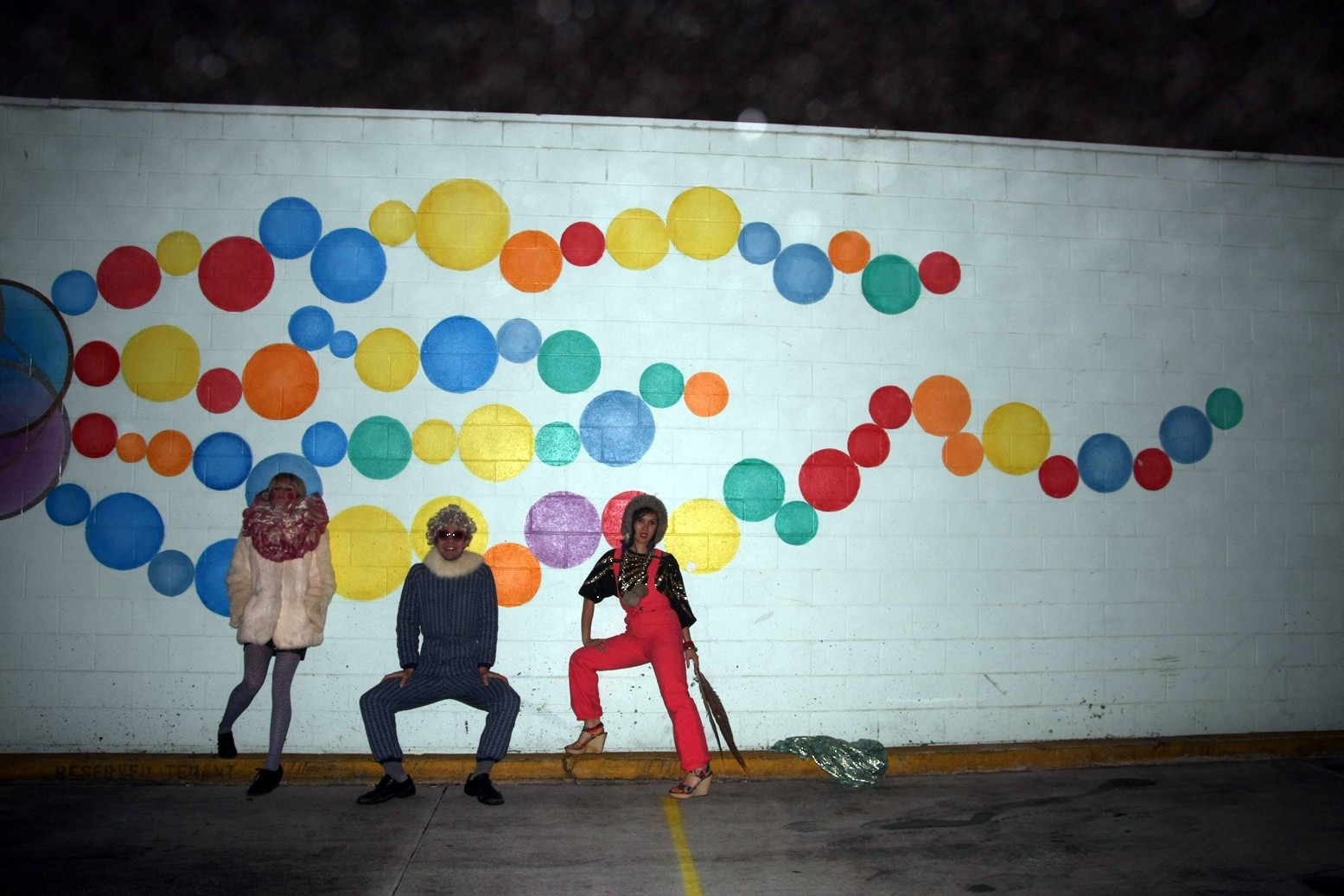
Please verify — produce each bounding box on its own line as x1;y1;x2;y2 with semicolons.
357;773;416;804
464;773;505;805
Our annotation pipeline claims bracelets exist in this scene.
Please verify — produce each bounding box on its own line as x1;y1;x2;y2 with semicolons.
683;641;697;651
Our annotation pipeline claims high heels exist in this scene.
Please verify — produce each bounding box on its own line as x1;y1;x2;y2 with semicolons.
218;731;238;759
247;764;283;796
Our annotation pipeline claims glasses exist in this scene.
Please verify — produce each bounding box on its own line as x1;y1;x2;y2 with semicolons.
437;528;466;542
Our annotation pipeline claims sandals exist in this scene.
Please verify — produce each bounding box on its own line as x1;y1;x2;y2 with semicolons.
669;763;713;799
565;722;607;755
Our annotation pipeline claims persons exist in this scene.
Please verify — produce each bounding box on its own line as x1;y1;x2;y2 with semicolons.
355;505;521;806
217;472;337;796
565;494;713;798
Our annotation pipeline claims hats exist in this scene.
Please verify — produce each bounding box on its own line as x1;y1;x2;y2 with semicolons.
620;495;668;545
